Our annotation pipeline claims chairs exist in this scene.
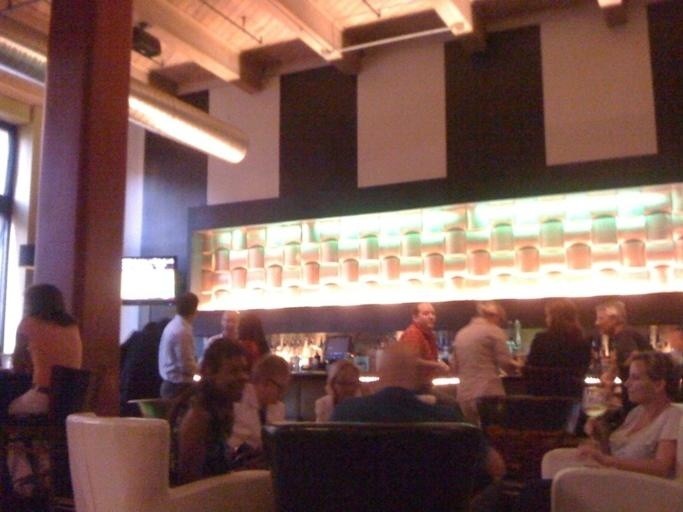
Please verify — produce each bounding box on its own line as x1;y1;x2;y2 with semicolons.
0;345;683;512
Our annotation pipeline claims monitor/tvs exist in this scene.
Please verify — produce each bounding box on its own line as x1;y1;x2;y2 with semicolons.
325;335;352;361
121;256;178;306
